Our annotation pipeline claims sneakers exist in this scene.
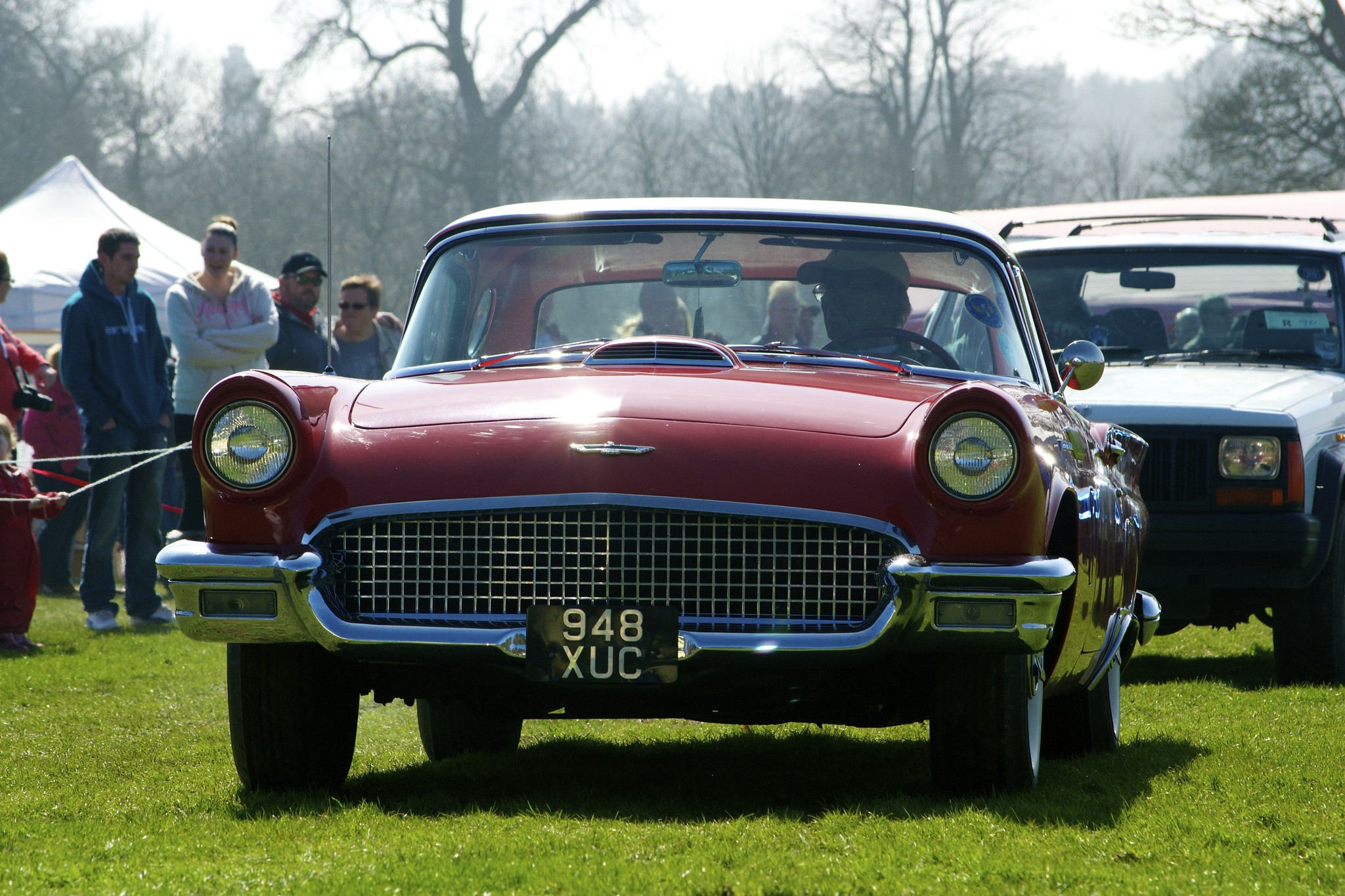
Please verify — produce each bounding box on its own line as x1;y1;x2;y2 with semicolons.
84;610;120;630
130;604;176;626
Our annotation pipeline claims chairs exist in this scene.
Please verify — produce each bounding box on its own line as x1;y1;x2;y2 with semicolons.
1101;306;1170;356
1241;306;1337;362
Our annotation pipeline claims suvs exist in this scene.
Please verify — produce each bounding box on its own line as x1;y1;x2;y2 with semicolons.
919;209;1344;686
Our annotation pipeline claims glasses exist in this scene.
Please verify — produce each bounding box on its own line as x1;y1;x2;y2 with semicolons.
1203;307;1228;316
0;276;18;287
813;282;889;302
284;272;324;286
336;300;371;312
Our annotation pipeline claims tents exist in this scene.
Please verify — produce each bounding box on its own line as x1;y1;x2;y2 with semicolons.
0;153;280;334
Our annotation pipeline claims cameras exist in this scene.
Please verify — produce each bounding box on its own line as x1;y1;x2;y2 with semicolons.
11;384;54;413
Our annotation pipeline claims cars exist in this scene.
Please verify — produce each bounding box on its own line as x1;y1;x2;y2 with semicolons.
153;195;1164;802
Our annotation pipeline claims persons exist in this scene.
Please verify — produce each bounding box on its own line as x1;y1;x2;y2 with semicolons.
58;228;176;632
535;294;570;350
22;343;89;598
1170;295;1251;353
796;237;951;369
611;280;692;338
332;273;404;379
750;279;821;348
164;213;281;527
0;254;58;464
264;252;404;373
0;413;69;653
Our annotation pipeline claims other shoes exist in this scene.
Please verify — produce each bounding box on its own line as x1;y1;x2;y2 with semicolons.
0;636;29;654
49;582;80;598
36;581;56;598
17;637;44;655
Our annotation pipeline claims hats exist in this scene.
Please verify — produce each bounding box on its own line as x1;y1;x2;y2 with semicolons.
280;252;328;280
797;238;911;290
801;300;821;315
